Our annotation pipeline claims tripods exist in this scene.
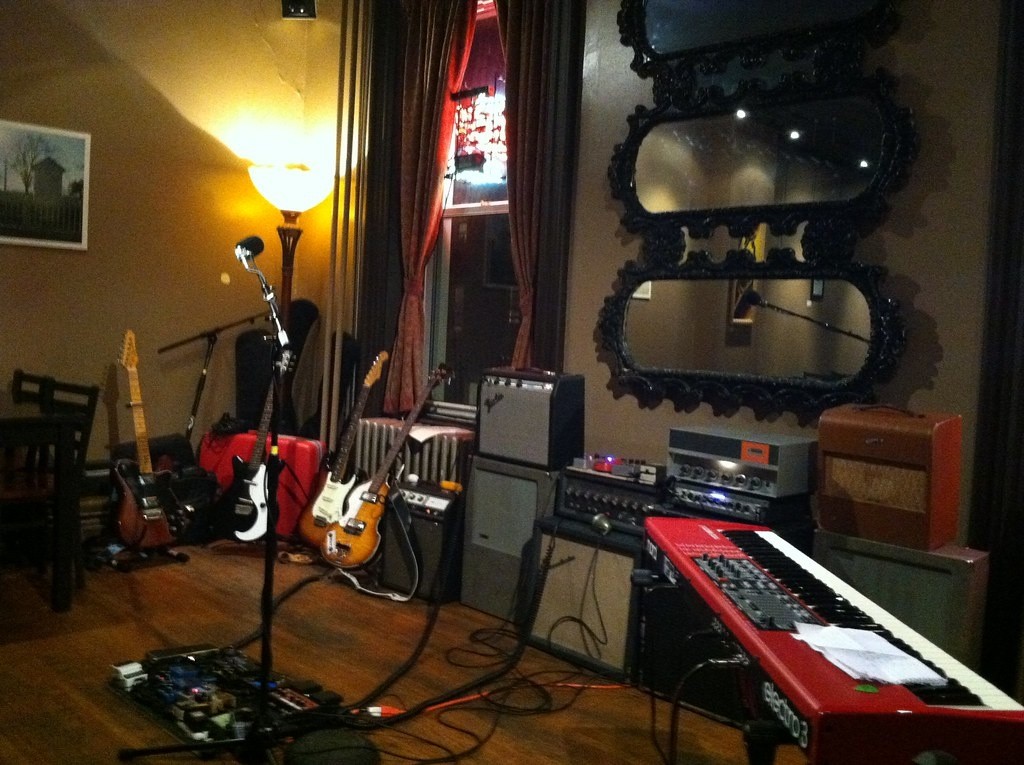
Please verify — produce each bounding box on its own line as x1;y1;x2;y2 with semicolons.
118;255;355;765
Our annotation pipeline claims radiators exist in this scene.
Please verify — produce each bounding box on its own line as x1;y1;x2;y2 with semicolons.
354;417;475;497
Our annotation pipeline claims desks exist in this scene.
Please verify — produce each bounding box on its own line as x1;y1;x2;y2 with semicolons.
0;399;90;613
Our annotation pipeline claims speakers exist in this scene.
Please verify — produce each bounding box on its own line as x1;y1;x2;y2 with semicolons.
817;404;962;552
522;516;652;689
473;366;585;471
381;482;468;604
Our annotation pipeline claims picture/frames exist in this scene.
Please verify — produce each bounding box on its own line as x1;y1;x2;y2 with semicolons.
730;279;753;325
0;118;91;251
810;279;825;301
633;280;652;299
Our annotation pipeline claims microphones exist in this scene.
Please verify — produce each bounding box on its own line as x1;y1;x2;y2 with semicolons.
234;236;264;272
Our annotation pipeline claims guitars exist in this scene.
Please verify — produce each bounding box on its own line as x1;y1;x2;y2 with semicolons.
295;349;389;545
323;362;455;571
218;346;298;544
112;329;187;550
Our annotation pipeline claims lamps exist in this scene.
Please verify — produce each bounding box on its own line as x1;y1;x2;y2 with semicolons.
443;72;507;188
247;161;336;435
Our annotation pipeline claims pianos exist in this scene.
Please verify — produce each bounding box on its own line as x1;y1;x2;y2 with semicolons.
643;516;1024;765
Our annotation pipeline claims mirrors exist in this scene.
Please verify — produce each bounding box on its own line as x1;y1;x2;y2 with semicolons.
595;1;923;428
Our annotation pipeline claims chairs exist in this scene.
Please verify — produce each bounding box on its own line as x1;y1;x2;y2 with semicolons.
1;369;100;588
